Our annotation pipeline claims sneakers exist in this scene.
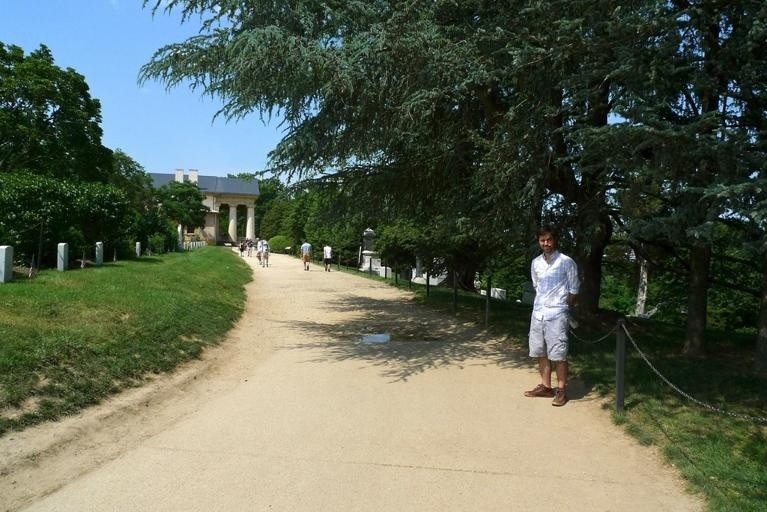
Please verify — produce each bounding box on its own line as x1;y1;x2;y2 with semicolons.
526;383;554;396
553;389;568;406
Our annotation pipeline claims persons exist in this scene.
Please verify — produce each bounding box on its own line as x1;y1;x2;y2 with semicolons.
324;242;332;271
524;229;581;406
239;238;270;267
301;239;312;270
